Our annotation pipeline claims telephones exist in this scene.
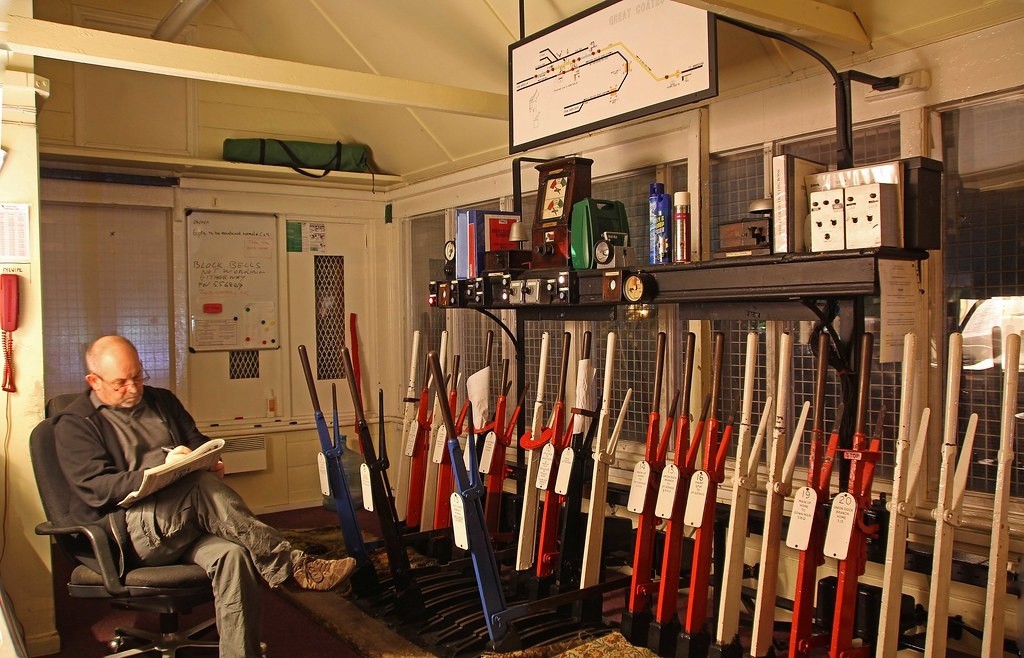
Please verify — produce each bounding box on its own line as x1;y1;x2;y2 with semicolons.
0;274;21;332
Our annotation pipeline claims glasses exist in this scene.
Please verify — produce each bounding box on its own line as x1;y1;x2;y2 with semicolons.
93;364;150;393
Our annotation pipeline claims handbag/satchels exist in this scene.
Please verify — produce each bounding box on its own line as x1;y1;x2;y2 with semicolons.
222;137;369;178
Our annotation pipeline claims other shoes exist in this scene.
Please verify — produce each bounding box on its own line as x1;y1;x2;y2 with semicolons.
283;550;357;593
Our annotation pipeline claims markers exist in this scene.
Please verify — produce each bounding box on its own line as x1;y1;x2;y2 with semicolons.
210;423;229;426
235;416;255;419
253;419;307;428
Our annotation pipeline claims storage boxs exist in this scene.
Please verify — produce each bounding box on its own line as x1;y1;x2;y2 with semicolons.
481;249;531;270
719;153;944;250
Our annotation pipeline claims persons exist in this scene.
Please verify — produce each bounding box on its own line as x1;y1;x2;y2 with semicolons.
53;334;358;658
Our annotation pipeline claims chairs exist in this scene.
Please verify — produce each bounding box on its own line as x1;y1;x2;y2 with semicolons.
29;419;268;658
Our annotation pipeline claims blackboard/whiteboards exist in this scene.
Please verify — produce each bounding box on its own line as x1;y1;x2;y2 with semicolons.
186;208;281;353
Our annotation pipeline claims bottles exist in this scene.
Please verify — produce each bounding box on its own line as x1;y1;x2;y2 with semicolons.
323;435;363;511
265;389;275;418
